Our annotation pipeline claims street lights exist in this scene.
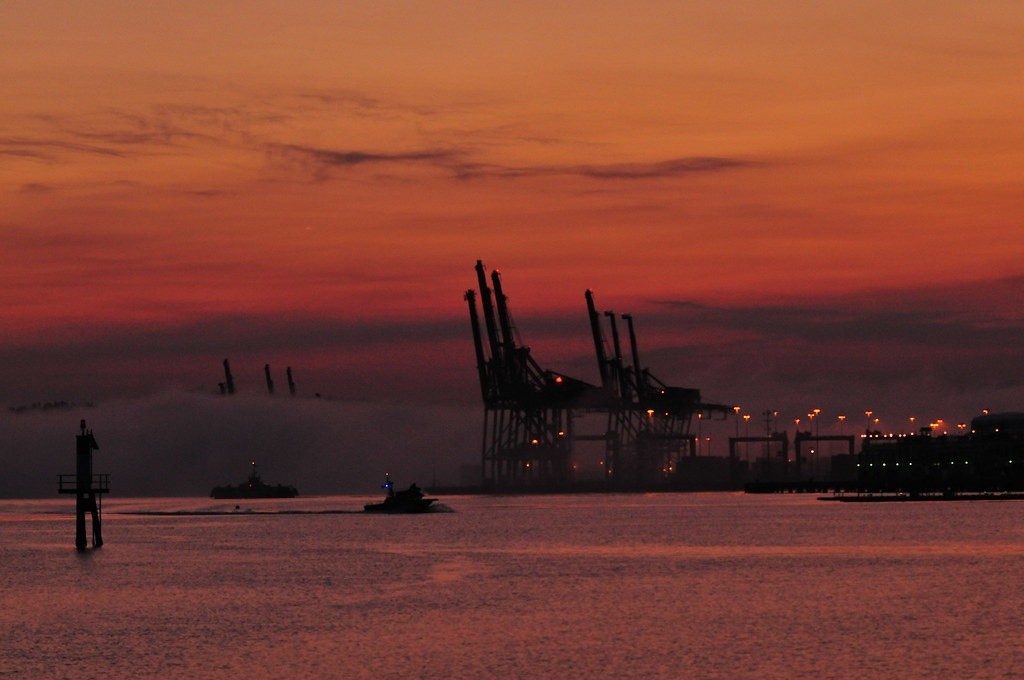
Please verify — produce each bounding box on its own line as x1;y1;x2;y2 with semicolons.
814;409;820;464
744;415;750;461
734;407;740;456
865;411;873;431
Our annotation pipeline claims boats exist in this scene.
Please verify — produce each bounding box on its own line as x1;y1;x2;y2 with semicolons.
363;476;438;514
210;470;299;499
